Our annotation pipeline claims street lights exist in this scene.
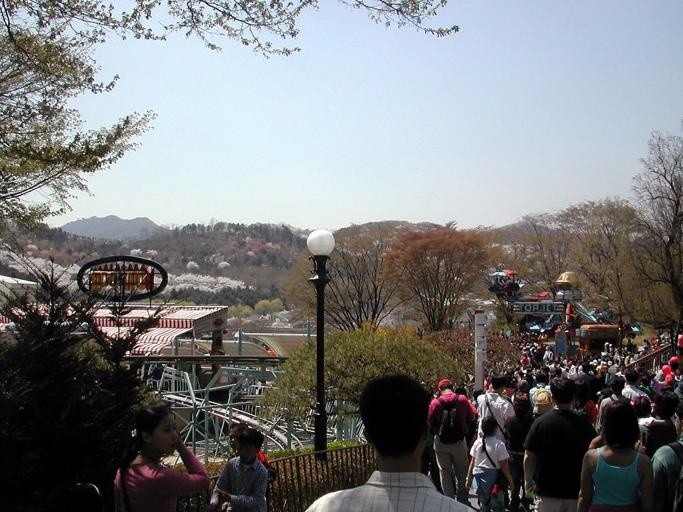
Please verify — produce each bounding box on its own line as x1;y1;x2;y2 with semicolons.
304;227;336;458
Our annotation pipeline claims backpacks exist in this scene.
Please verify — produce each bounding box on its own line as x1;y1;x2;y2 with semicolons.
536;387;553;413
437;395;467;444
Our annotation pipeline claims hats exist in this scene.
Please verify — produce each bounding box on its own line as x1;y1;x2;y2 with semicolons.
438;380;452;388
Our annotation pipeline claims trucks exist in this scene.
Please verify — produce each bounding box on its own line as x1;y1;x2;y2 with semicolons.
578;325;619;356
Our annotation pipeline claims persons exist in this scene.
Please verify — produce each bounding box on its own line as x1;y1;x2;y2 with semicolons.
452;324;683;497
207;428;269;512
226;421;276;512
576;396;654;511
428;379;474;507
110;401;211;511
647;396;683;512
502;395;537;511
299;371;480;512
521;377;596;511
474;375;515;508
463;415;516;512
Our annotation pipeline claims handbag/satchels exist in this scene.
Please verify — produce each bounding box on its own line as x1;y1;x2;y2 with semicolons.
497;469;509;490
